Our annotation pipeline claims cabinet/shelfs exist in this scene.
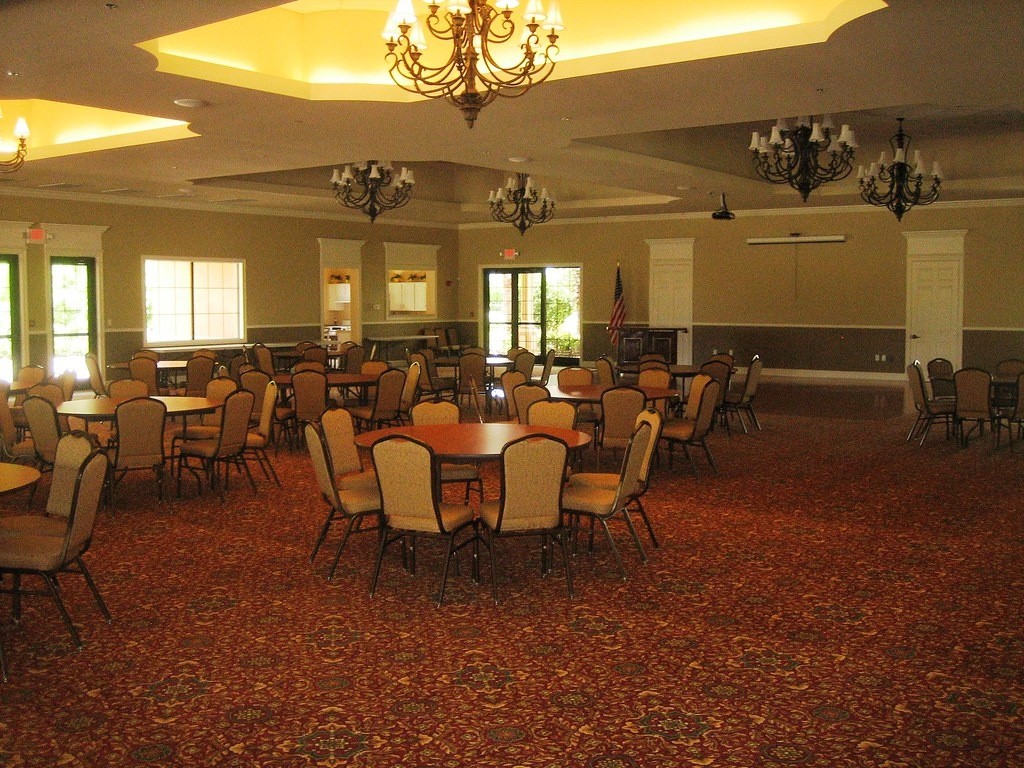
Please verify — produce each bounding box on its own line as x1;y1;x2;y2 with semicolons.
326;284;353;302
388;282;427;312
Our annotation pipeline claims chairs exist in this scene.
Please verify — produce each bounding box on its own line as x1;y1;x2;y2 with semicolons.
568;405;665;557
408;395;484;506
926;357;956;400
526;396;579;482
953;366;1013;451
547;420;653;582
0;324;766;487
992;359;1024;439
905;359;956;448
0;428;96;594
0;447;114;652
302;418;409;582
368;433;478;610
106;395;168;506
478;432;575;606
21;395;63;511
318;404;385;547
177;388;258;507
995;371;1024;454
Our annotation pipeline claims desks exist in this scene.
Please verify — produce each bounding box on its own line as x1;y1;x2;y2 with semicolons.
271;350;347;368
353;422;594;460
544;384;680;410
427;356;515;378
50;396;226;490
365;335;439;362
0;462;43;517
106;360;221;390
614;364;704;383
927;373;1019;386
8;380;38;396
268;372;379;405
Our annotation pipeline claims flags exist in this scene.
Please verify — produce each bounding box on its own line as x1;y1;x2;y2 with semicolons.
608;268;625;346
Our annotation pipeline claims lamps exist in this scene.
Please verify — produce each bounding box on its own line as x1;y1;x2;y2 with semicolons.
486;172;556;236
747;112;860;205
0;117;32;174
329;160;416;225
379;0;566;130
856;116;947;224
